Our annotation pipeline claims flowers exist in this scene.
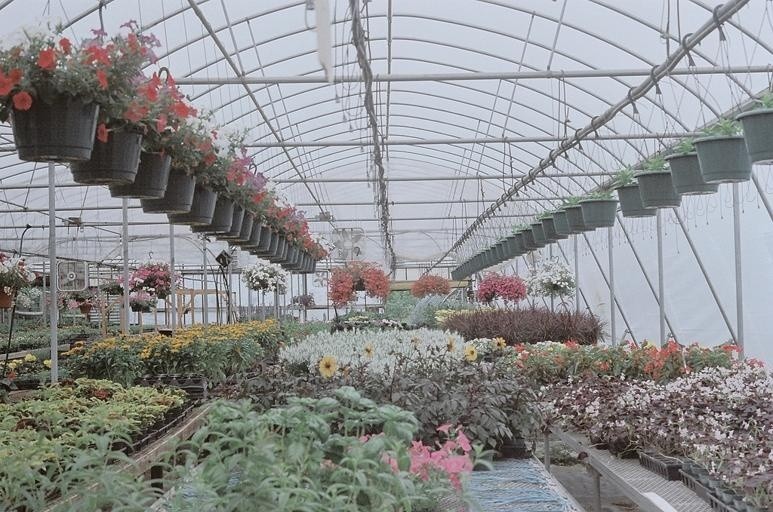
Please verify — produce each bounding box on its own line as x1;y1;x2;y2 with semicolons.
0;19;331;262
0;258;773;512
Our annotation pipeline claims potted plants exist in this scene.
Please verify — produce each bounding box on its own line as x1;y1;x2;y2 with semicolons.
450;3;773;281
